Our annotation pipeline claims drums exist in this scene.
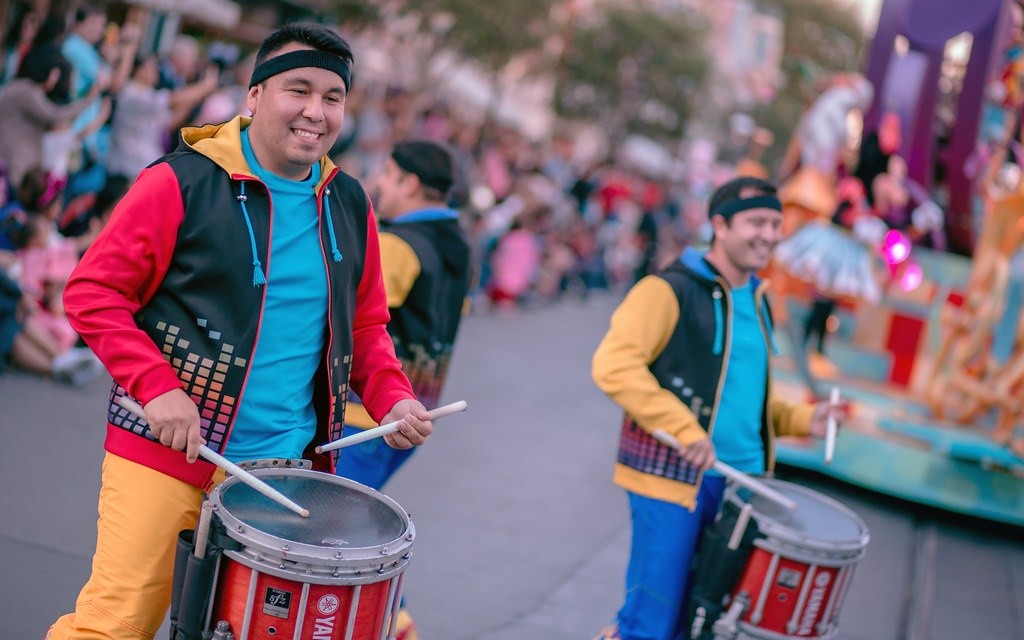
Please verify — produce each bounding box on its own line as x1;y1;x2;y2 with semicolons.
175;456;415;639
692;478;870;640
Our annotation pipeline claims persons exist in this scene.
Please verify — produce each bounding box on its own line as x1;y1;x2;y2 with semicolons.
333;138;472;492
591;175;851;640
0;0;1022;396
39;22;435;640
